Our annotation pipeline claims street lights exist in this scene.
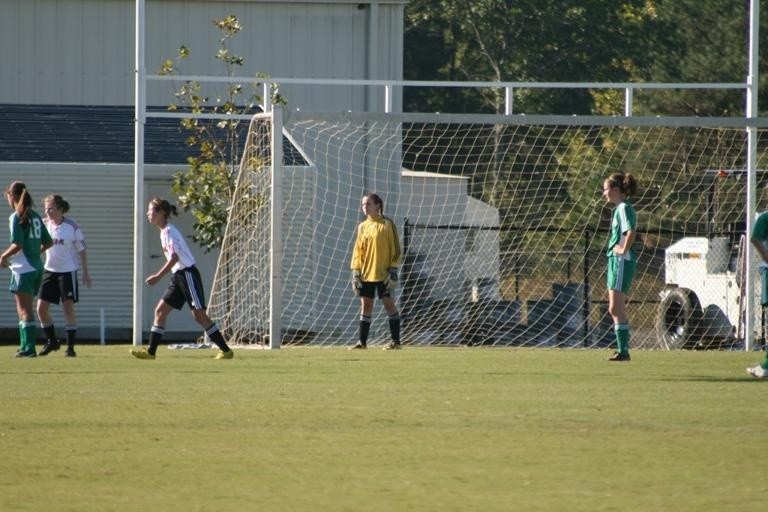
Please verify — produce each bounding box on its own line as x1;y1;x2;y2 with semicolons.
562;245;574;283
513;248;521;299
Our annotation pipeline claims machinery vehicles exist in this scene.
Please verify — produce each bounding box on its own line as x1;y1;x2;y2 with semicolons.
656;169;768;350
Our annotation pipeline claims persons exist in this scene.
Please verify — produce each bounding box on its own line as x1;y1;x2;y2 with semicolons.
0;180;54;358
351;192;402;350
602;172;637;361
745;213;767;381
129;197;233;361
37;193;92;356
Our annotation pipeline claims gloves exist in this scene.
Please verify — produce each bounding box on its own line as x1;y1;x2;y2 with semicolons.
383;267;399;291
351;270;363;296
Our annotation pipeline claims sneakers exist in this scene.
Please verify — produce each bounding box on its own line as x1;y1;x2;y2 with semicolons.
607;350;631;361
215;349;233;359
382;340;402;350
746;364;768;378
65;348;77;357
38;341;61;356
349;340;367;350
129;348;155;360
15;349;37;358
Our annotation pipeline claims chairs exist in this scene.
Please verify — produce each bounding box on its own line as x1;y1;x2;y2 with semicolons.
728;220;746;252
